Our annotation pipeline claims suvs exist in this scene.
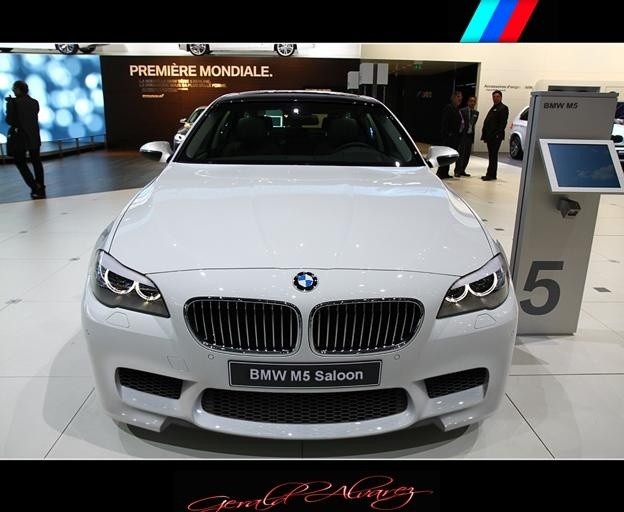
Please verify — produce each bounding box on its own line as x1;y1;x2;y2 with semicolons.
507;105;623;174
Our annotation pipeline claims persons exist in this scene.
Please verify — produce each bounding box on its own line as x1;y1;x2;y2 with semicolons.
436;91;463;179
480;90;508;181
3;80;45;200
453;95;478;177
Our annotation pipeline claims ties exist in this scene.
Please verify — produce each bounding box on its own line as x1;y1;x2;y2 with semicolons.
470;110;473;125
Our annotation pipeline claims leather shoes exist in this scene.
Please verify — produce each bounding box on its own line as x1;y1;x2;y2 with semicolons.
436;171;470;179
481;176;495;181
31;181;46;199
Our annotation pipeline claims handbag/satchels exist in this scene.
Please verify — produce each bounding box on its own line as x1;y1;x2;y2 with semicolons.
6;99;28;156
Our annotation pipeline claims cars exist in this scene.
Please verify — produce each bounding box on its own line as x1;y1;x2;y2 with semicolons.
79;88;516;445
170;102;211;152
0;43;109;54
177;43;302;57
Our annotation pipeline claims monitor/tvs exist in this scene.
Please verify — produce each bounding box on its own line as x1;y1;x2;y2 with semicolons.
538;138;624;194
